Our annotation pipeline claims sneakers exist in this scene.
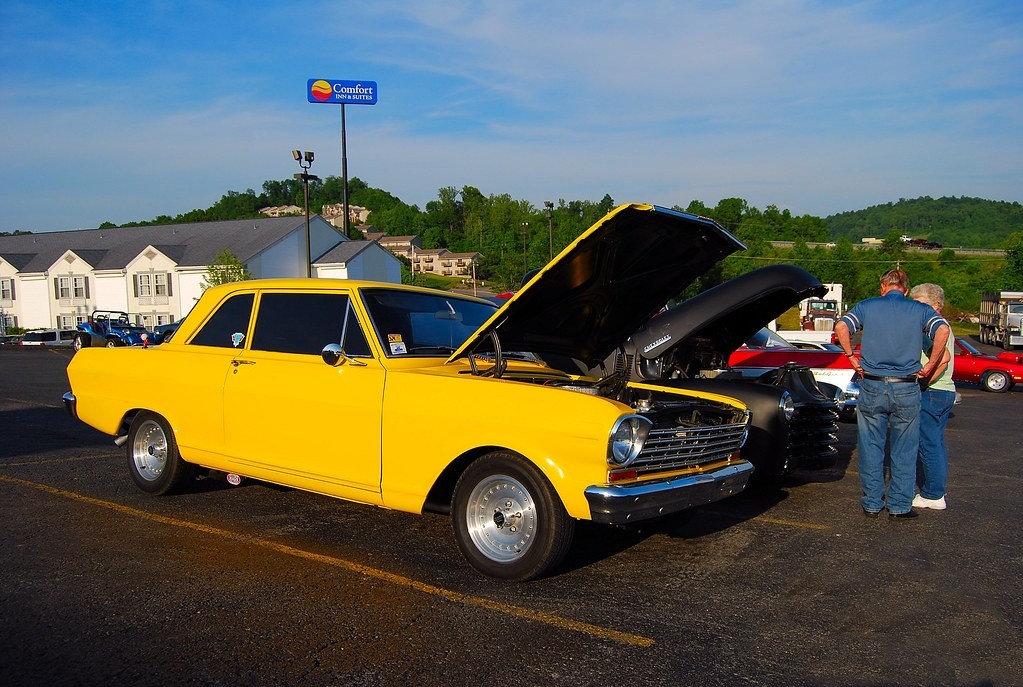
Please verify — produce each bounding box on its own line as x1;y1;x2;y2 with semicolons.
912;493;947;510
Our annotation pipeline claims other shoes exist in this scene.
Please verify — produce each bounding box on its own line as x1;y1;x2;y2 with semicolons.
888;508;922;519
864;508;879;517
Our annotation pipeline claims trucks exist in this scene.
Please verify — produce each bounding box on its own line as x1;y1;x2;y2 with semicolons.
979;288;1023;351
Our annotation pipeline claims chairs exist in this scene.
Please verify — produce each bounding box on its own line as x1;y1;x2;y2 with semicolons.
97;314;108;332
118;316;126;324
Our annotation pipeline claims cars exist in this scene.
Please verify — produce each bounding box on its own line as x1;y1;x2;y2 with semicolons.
0;328;79;351
899;233;943;251
72;310;161;355
826;241;841;249
468;262;841;480
952;334;1023;394
154;317;186;344
64;201;756;586
649;283;862;422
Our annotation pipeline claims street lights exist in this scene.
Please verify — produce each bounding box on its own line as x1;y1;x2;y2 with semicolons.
292;150;318;280
521;221;530;274
544;201;555;263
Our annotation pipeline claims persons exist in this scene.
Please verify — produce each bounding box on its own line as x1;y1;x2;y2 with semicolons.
908;283;957;511
835;268;950;520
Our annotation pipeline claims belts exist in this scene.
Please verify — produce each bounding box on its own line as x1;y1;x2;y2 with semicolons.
863;373;917;383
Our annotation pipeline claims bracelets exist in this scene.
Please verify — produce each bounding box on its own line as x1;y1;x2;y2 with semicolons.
846;352;854;357
